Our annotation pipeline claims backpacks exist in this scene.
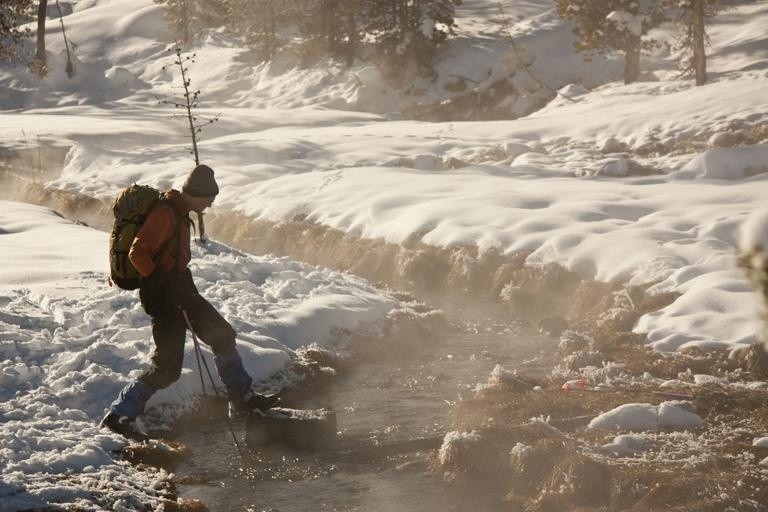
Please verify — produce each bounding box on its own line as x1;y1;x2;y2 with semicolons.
110;183;181;292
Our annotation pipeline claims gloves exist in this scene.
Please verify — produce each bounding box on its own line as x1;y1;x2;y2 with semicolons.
149;268;184;304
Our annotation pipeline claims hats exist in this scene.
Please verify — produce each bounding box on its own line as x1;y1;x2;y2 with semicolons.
183;164;219;197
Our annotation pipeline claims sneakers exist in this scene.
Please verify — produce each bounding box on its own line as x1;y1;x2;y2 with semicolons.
100;410;151;443
227;390;279;419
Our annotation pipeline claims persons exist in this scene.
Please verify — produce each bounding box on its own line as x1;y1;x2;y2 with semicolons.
97;164;283;441
